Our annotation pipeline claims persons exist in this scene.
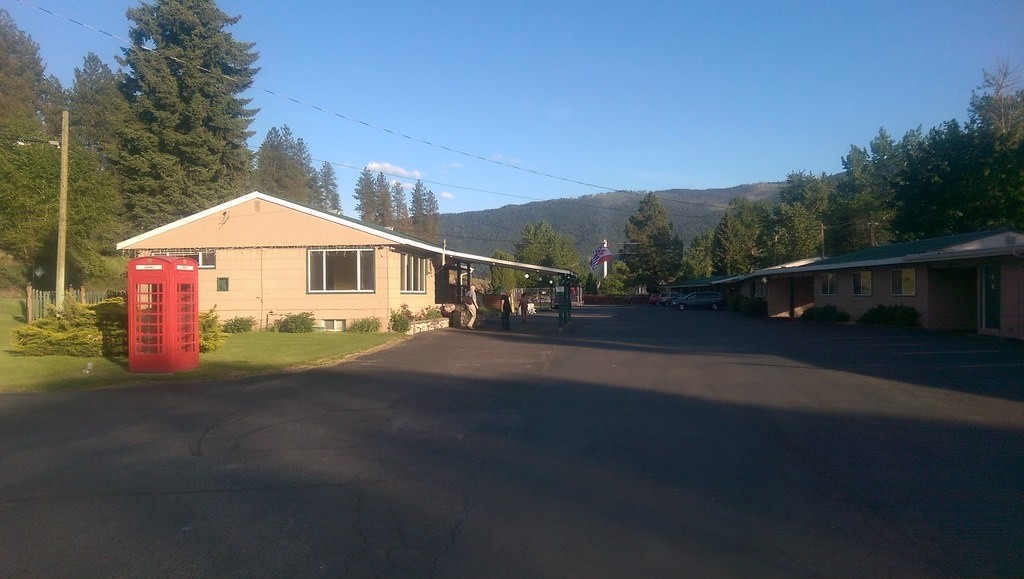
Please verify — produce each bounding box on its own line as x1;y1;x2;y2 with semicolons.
500;290;511;330
465;285;479;330
519;294;527;324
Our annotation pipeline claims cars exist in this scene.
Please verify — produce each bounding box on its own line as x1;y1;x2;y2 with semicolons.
649;292;681;307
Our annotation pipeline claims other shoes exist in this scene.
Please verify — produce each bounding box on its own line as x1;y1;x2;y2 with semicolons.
466;325;472;330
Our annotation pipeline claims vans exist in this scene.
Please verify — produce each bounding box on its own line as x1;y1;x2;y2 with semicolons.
674;291;724;311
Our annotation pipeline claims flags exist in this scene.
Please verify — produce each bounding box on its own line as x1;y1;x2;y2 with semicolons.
589;244;613;271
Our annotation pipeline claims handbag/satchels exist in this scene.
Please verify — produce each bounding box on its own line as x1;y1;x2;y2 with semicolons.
465;296;473;305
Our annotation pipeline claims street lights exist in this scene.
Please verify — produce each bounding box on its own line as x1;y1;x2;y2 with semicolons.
550;280;553;308
538;277;542;309
